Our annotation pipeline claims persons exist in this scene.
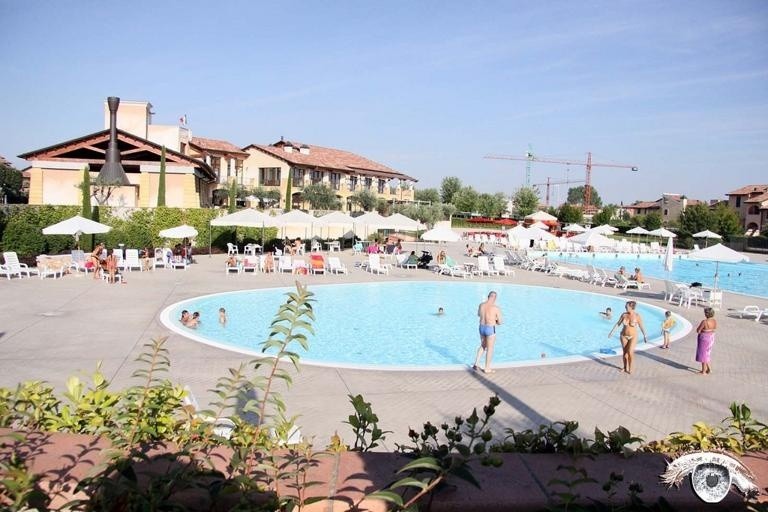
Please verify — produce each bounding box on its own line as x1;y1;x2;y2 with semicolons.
219;308;227;328
696;308;718;375
607;300;648;374
432;308;446;317
186;312;200;328
597;306;613;322
471;290;502;374
179;309;190;325
660;311;673;349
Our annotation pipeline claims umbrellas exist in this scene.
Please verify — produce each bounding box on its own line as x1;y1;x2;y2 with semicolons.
41;214;115;249
506;210;750;310
157;223;199;259
420;227;462;265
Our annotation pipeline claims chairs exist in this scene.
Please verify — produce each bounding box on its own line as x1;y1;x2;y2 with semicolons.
517;253;768;324
221;235;515;279
1;245;189;281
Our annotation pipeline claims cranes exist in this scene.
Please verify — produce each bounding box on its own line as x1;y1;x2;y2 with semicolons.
480;150;639;216
